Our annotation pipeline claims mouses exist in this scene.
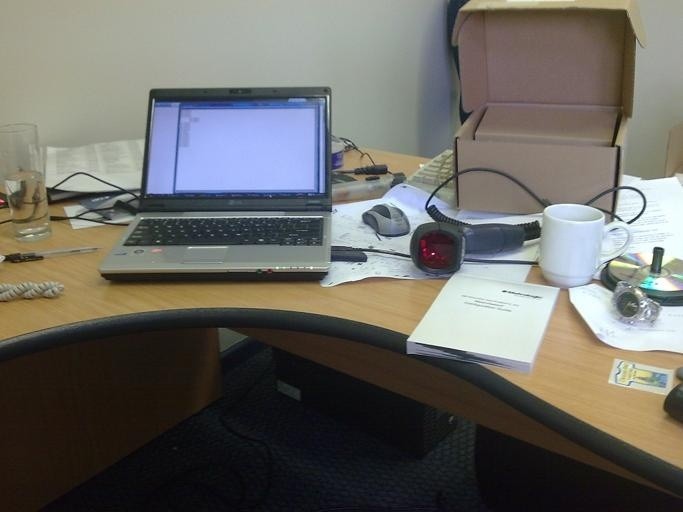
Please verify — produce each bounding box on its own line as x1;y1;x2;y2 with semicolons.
360;205;411;238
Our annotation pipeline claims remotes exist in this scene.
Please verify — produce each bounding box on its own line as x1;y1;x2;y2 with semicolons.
331;246;366;262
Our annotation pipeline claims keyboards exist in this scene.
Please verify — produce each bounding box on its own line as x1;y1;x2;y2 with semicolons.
404;149;454;206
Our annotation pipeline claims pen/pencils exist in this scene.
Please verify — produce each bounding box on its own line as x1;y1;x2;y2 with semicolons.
0;247;101;262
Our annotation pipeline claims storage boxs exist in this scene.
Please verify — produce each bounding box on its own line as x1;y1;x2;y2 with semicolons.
445;0;648;223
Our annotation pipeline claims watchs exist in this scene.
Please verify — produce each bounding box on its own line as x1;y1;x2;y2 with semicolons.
615;281;660;325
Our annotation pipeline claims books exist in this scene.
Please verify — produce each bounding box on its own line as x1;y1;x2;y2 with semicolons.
405;274;561;370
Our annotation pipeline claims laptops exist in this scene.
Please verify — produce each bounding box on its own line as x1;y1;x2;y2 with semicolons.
95;89;331;283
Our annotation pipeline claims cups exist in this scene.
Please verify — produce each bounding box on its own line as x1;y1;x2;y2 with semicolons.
0;123;53;243
540;204;632;289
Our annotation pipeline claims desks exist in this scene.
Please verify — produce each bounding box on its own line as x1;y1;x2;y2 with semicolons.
1;141;682;512
